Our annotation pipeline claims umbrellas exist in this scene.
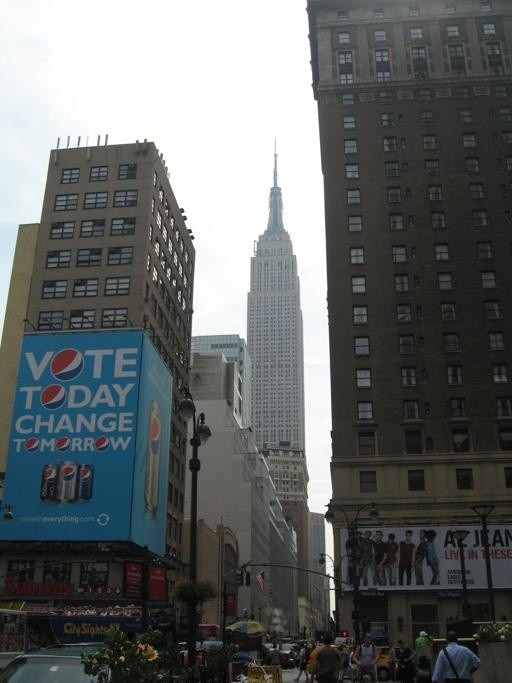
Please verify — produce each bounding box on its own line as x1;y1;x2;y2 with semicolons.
225;620;268;656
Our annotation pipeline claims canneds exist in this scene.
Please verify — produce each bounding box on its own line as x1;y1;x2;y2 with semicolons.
40;460;93;502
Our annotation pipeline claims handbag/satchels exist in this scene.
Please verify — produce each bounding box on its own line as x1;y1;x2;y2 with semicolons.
457;678;473;683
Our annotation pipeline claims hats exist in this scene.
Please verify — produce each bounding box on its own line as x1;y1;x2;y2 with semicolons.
420;630;428;635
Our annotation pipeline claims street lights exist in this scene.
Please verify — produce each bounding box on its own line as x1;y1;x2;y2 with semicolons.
318;551;353;636
239;502;381;645
175;386;212;682
451;503;498;625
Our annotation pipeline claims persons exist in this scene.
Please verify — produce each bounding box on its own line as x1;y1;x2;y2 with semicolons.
345;529;440;586
270;630;480;683
